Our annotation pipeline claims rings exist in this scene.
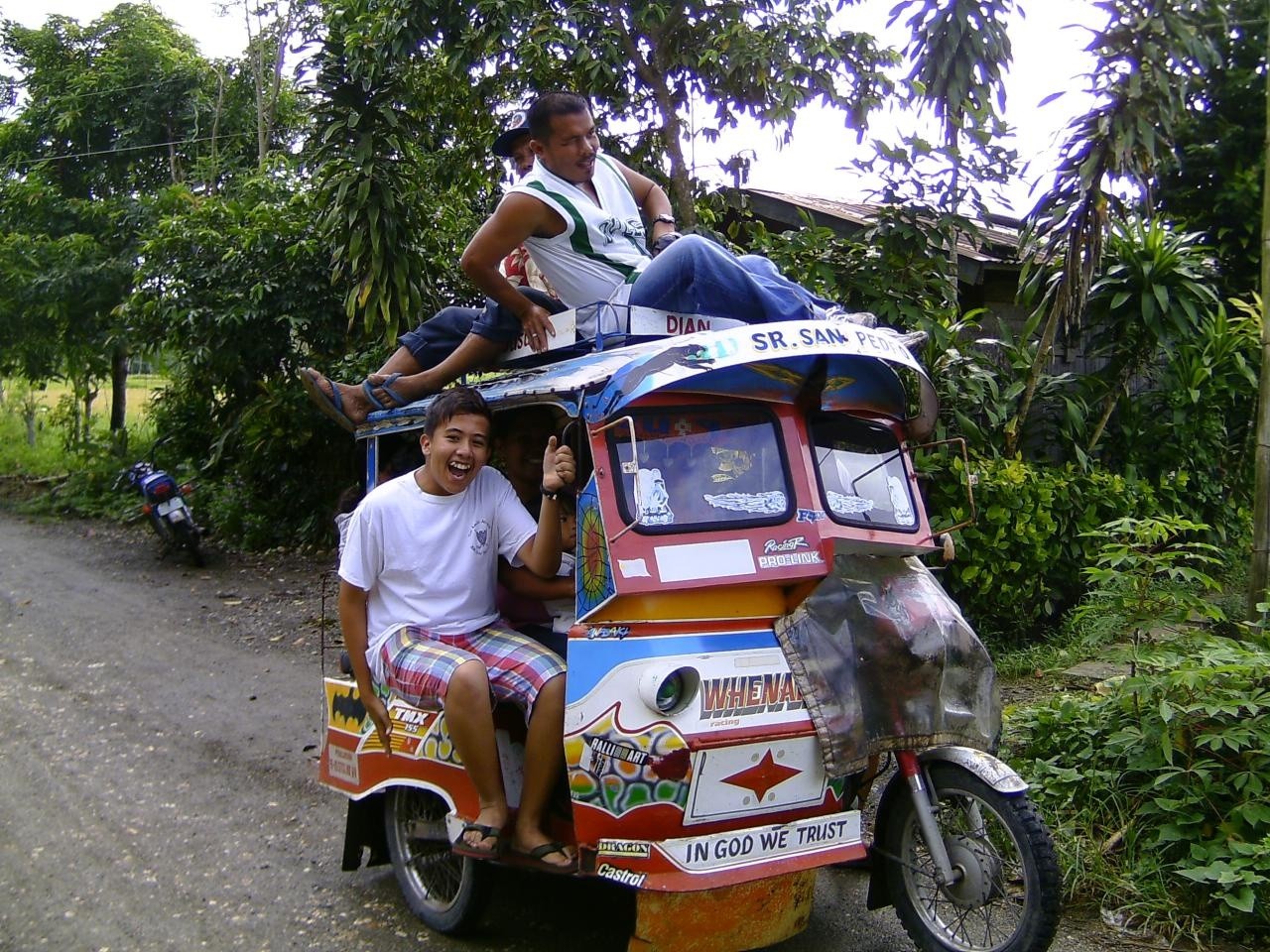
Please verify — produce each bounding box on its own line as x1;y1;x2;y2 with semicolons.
533;333;537;337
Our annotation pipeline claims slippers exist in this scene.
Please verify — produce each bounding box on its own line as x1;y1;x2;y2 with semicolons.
510;835;579;868
299;366;357;434
362;372;422;410
462;813;513;855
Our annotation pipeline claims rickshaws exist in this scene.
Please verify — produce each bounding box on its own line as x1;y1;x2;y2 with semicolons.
319;299;1063;952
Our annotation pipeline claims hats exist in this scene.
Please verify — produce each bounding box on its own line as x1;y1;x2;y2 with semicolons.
491;112;530;158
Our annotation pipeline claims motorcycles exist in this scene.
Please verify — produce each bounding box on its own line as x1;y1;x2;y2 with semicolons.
110;430;209;571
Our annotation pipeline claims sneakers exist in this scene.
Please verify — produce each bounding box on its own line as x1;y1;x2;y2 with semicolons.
877;327;928;357
821;304;878;331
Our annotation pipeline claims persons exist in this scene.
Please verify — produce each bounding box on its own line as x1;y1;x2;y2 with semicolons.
338;386;579;874
301;110;569;431
462;91;928;359
335;447;396;561
493;406;577;663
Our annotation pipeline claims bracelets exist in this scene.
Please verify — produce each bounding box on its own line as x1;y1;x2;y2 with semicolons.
651;214;675;227
539;483;565;500
